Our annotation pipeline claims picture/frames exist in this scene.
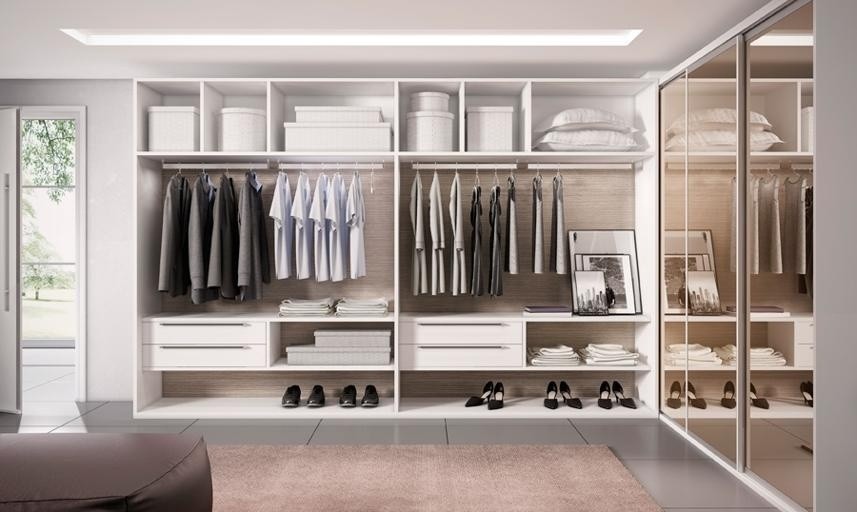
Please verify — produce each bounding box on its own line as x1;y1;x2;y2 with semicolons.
566;228;643;316
661;226;724;317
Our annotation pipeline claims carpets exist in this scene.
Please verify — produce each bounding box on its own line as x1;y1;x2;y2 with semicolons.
203;445;667;512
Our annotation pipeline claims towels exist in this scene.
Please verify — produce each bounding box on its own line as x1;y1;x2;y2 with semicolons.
526;340;637;367
665;344;784;370
277;295;391;320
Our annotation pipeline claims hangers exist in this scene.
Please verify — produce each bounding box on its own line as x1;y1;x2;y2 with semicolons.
734;168;813;186
404;159;564;195
160;163;377;195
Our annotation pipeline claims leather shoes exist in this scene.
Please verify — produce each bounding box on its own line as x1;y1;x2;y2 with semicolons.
360;385;379;407
339;385;356;407
306;385;325;407
282;385;301;407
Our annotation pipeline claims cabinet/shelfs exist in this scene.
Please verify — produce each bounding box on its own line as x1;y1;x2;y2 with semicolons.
398;79;659;157
658;71;814;161
133;79;395;162
397;312;654;415
664;316;816;418
133;304;396;419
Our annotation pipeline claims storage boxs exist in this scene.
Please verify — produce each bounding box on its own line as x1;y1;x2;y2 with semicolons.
408;112;456;151
213;105;268;154
143;104;198;153
292;104;384;121
800;107;813;154
281;121;392;152
464;103;515;151
409;91;450;113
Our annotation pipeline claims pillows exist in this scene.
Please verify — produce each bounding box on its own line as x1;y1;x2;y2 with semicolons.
529;132;637;152
532;105;637;137
665;131;784;153
666;107;771;134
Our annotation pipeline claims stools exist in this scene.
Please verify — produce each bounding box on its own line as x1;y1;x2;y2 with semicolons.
0;429;215;512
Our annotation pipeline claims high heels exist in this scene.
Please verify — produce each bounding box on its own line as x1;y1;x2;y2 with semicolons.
721;381;736;409
806;381;812;393
667;381;681;409
544;381;558;409
464;381;493;407
750;382;769;409
611;381;636;409
487;382;504;410
598;381;612;409
560;381;582;409
683;381;706;409
799;382;813;407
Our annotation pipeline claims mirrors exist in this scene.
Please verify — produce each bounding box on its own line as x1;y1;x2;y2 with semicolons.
658;2;819;512
